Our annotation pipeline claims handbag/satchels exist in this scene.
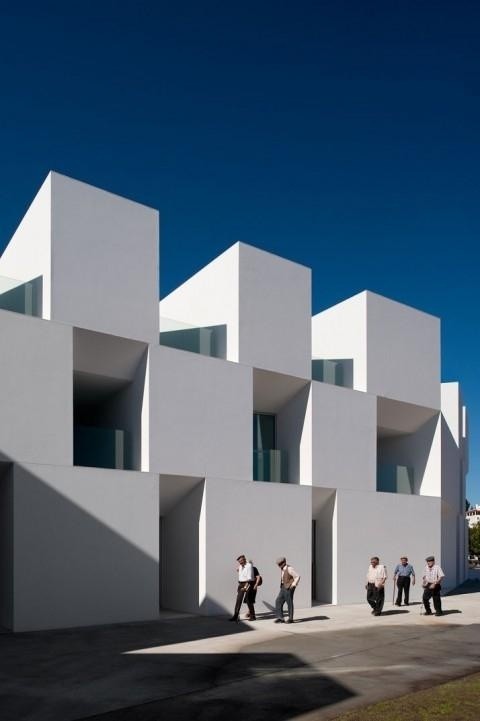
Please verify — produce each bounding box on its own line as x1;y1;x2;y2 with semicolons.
254;576;262;586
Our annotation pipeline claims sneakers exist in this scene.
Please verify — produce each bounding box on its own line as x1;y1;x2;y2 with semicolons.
425;612;443;616
372;611;382;616
228;614;256;621
274;619;293;623
395;601;410;606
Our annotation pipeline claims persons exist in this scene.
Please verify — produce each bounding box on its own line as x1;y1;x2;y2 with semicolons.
228;556;257;621
364;557;387;616
422;556;446;616
392;556;416;606
244;560;261;617
273;556;300;623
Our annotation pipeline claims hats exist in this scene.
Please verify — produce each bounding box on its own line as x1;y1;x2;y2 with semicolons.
276;558;285;564
425;556;434;561
